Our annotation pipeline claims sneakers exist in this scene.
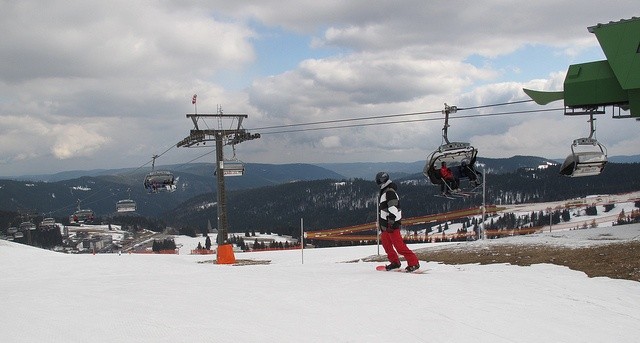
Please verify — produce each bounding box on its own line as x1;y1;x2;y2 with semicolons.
453;189;458;192
448;190;453;193
405;262;420;273
441;193;447;196
457;188;462;192
385;262;401;271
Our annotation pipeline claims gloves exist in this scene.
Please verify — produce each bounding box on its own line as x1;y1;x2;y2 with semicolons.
387;216;395;224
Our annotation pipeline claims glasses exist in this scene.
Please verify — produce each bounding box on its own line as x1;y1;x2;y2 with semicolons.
377;176;388;182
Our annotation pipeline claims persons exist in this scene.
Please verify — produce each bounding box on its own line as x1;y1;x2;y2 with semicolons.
375;171;420;272
430;163;451;196
460;161;468;177
440;161;462;193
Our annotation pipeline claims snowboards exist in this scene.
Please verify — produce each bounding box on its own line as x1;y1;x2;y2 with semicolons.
376;266;432;274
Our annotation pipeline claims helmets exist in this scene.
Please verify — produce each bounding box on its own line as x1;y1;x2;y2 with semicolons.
376;172;389;186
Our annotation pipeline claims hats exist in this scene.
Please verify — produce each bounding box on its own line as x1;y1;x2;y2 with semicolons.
433;163;437;166
